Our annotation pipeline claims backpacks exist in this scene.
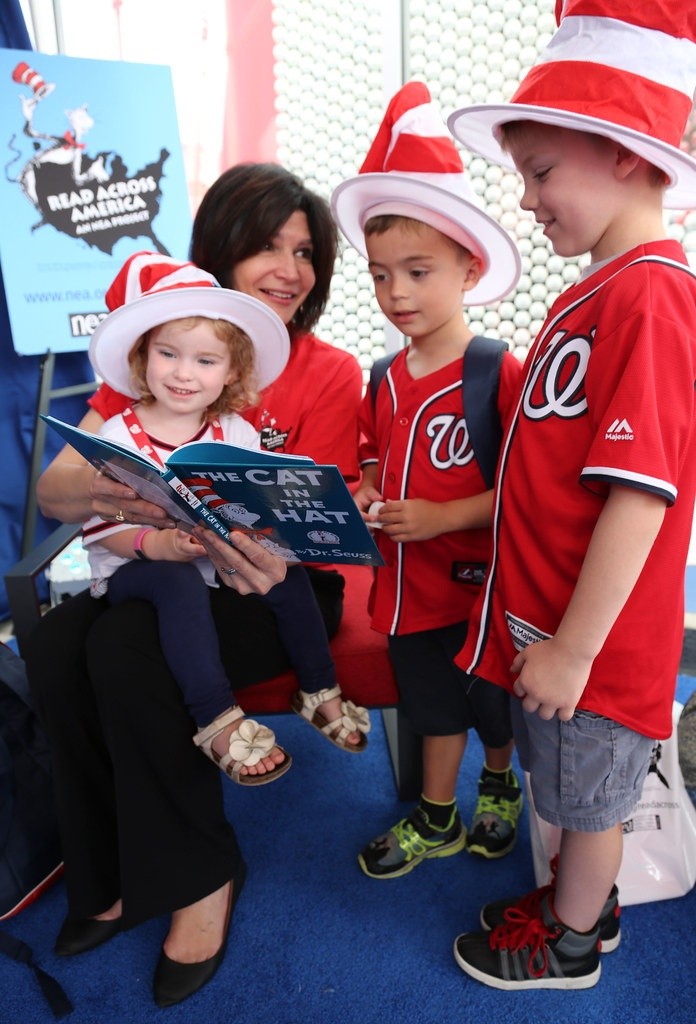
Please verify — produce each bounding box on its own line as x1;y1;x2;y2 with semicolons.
0;643;69;921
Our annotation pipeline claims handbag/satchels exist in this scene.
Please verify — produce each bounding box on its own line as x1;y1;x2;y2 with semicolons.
524;673;696;907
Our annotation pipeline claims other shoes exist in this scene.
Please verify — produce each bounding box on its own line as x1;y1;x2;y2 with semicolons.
54;913;125;956
153;861;247;1008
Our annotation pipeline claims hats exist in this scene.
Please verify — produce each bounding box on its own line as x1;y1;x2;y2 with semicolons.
447;0;696;211
89;251;291;400
330;81;521;306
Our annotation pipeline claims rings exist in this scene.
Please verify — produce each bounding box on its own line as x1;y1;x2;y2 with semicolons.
221;569;237;575
116;510;125;521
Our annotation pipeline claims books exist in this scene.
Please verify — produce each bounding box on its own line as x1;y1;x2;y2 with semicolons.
40;413;388;567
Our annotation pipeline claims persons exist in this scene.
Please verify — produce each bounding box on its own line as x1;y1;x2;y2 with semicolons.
445;1;696;990
329;78;524;879
82;251;371;786
22;158;364;1007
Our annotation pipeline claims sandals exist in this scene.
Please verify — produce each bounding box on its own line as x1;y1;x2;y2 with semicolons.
290;684;371;752
192;705;293;787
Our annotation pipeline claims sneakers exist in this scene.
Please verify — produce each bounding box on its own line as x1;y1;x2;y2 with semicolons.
453;890;601;990
479;854;621;952
357;806;468;879
466;774;523;859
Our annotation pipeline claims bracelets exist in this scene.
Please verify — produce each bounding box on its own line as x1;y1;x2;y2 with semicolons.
133;528;155;561
